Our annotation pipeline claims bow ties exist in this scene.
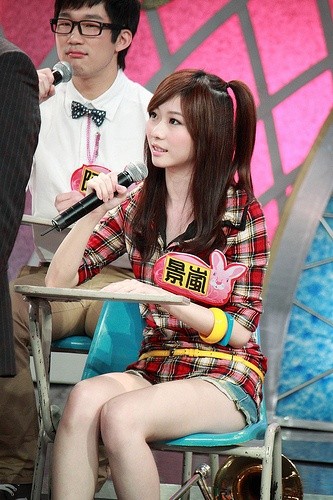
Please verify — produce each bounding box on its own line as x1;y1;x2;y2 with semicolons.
71;100;107;128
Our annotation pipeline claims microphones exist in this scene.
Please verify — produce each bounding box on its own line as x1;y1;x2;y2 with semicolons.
51;159;149;232
51;61;73;86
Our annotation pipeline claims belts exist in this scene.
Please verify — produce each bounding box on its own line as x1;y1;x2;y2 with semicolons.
138;348;265;384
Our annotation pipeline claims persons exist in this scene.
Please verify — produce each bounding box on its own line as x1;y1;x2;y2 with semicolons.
45;69;268;500
0;0;155;500
0;38;41;377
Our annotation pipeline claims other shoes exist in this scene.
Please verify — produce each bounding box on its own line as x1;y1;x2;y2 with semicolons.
0;483;35;500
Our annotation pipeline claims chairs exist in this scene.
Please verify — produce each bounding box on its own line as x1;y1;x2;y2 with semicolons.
16;284;283;500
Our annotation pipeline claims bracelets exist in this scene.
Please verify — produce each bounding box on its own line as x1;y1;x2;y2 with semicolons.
199;308;234;346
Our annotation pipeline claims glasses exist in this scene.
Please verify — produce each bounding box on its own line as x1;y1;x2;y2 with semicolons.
50;18;122;36
